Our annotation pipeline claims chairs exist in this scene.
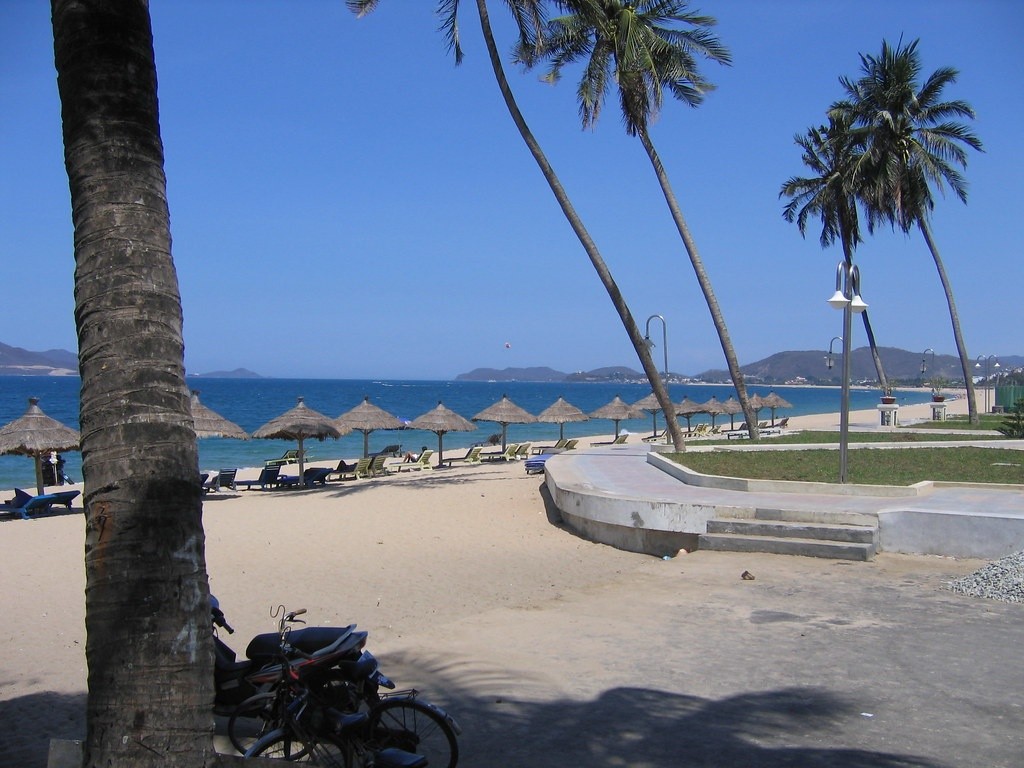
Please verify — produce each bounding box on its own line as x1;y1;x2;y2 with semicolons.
485;444;516;460
470;432;503;447
388;449;434;472
1;494;58;519
199;444;401;498
530;418;790;456
47;490;81;512
514;441;531;460
440;447;483;467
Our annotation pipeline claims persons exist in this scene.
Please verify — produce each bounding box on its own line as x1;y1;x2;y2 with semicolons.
402;446;427;463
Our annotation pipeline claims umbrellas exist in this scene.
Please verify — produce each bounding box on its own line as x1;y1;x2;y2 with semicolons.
338;396;408;458
191;390;250;440
407;403;478;465
631;390;681;436
472;397;538;452
589;396;647;438
0;397;81;496
676;396;712;431
537;397;589;440
385;416;411;454
252;396;353;487
703;391;792;430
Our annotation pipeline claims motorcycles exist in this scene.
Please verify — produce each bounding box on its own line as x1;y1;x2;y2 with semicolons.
208;588;396;740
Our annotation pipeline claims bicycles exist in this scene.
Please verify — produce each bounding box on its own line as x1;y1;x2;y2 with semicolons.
226;603;462;768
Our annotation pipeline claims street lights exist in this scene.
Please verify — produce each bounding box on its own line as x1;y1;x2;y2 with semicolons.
975;354;988;413
641;314;673;445
987;354;1000;412
918;347;936;402
824;260;869;490
